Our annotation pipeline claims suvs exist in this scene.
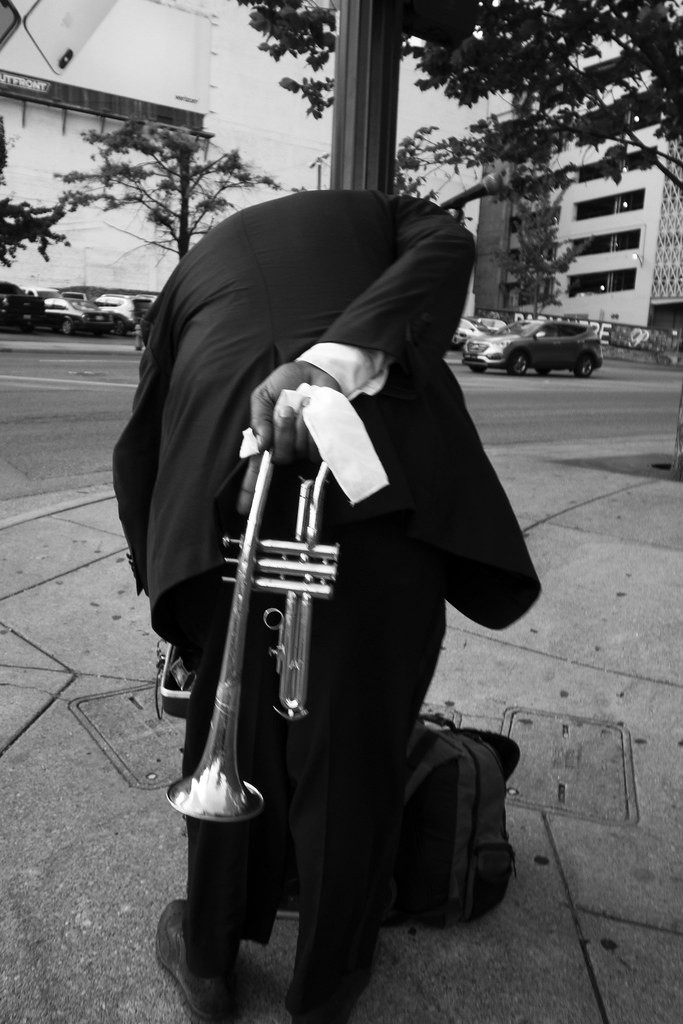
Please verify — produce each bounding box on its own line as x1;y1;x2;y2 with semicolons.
462;320;605;378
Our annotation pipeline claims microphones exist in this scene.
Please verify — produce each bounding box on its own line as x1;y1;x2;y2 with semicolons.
440;174;502;212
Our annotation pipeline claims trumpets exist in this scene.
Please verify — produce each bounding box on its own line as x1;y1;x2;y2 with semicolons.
165;446;340;824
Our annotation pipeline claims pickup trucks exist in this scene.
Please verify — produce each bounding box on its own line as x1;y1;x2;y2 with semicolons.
0;281;46;332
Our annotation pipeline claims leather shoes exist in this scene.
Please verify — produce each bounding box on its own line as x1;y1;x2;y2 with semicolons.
156;900;233;1024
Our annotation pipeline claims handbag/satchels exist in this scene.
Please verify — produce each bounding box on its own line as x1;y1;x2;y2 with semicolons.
278;714;519;928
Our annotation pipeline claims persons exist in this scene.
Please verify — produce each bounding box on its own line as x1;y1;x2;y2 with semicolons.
112;189;541;1024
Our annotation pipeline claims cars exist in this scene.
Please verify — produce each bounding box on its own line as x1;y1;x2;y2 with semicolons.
21;286;160;338
474;317;523;336
452;317;489;351
